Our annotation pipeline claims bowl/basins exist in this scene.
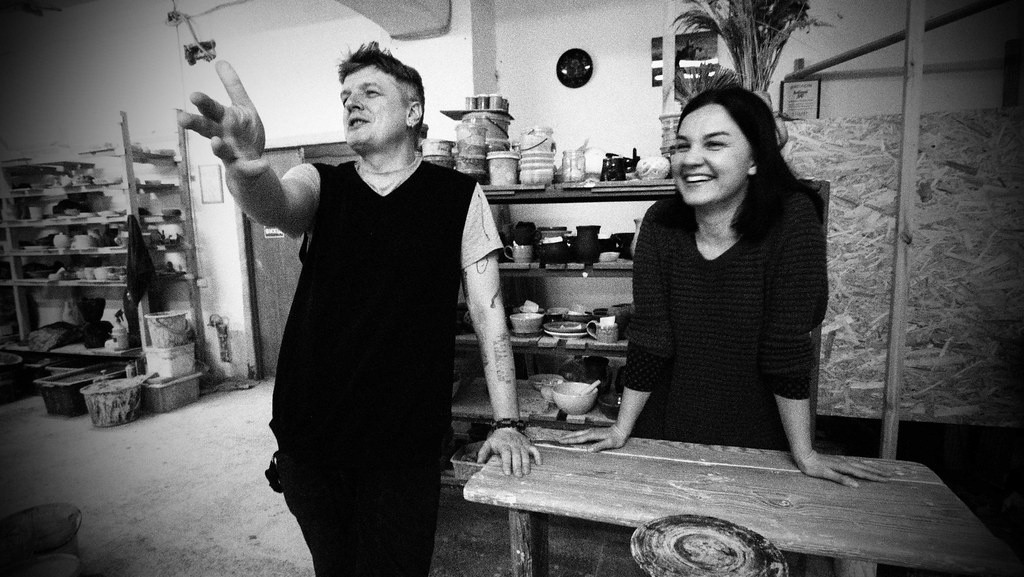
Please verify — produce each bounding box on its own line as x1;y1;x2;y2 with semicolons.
551;382;598;415
80;379;142;427
509;313;544;333
598;394;622;420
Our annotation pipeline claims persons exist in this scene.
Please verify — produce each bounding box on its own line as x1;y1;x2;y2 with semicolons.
555;83;895;489
174;41;543;577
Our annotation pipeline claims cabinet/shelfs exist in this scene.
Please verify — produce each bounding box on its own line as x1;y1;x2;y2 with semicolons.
1;108;205;361
440;180;675;484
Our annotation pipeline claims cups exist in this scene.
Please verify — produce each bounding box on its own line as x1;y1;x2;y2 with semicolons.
562;150;586;183
486;151;520;186
587;320;619;343
28;206;43;219
503;245;533;263
462;95;511;112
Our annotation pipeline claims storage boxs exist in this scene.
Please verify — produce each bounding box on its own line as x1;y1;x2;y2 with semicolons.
141;343;202;413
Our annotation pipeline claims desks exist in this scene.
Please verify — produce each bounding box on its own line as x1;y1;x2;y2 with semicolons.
462;426;1024;577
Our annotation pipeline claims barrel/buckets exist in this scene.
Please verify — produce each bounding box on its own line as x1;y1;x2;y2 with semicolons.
461;113;510;141
143;311;188;348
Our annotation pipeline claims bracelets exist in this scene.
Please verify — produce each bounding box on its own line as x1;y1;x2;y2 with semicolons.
492;418;526;432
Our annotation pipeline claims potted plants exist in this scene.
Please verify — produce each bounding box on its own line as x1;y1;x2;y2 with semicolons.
668;0;842;112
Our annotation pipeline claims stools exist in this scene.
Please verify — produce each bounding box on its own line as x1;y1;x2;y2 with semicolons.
631;514;790;577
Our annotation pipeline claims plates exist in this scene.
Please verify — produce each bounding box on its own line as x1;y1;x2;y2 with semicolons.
543;321;589;338
